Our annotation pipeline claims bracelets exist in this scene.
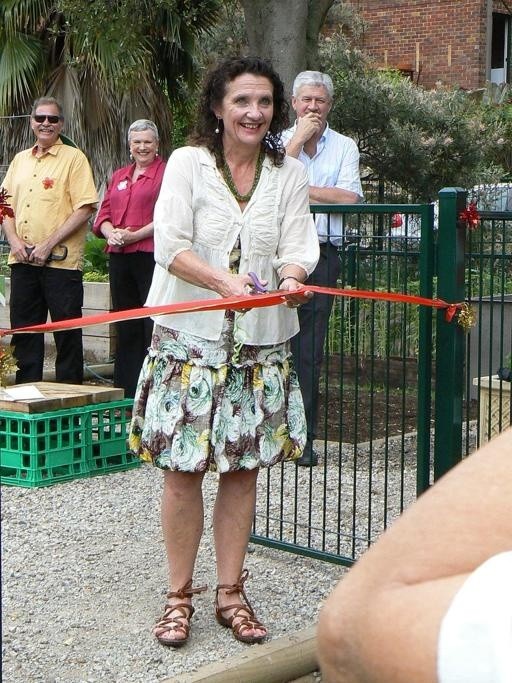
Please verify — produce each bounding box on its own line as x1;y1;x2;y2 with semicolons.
278;276;298;289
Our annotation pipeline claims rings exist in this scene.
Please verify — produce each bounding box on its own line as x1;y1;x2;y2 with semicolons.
38;262;42;264
293;304;300;308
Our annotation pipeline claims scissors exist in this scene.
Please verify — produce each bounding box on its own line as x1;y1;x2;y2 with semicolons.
248;272;284;296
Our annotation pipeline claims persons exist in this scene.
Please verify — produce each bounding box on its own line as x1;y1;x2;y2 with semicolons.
317;428;512;683
92;119;166;398
0;97;100;383
128;58;320;645
269;70;365;467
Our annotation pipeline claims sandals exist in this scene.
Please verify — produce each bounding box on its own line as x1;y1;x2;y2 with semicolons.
154;579;195;646
214;569;268;643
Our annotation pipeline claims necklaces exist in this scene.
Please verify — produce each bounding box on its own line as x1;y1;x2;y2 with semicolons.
221;150;265;202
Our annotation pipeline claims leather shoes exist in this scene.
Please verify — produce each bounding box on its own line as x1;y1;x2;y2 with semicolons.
298;436;318;466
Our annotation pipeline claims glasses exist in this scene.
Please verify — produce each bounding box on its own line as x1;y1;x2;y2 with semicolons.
31;115;63;123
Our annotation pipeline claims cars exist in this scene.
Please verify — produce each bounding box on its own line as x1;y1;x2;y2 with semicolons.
385;182;512;251
